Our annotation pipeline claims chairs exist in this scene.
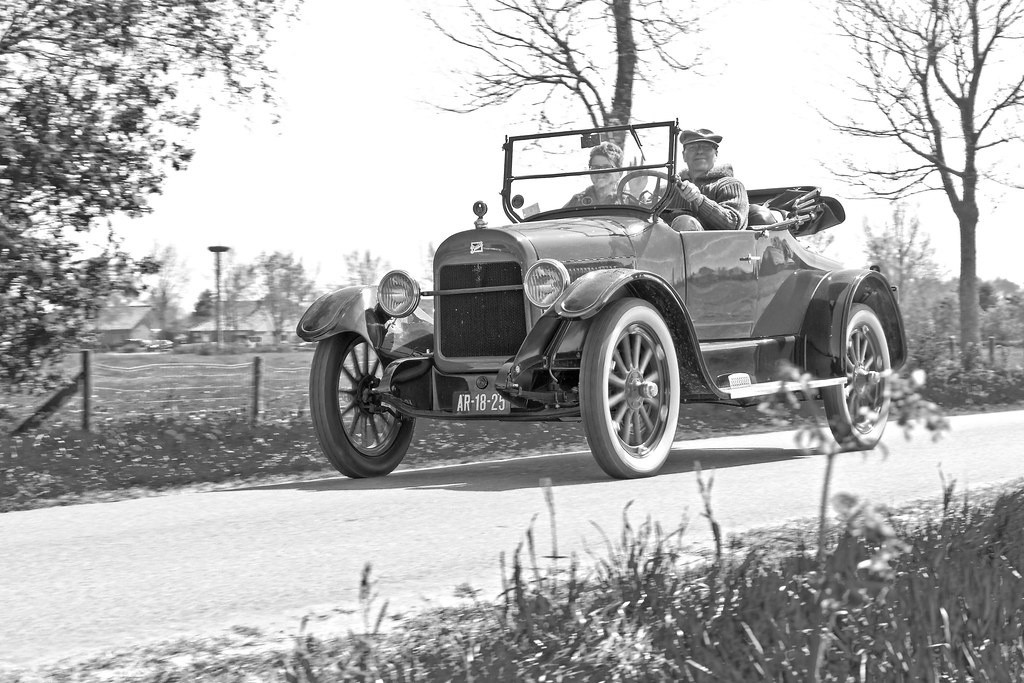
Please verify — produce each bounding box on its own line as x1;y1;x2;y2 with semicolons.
747;204;777;227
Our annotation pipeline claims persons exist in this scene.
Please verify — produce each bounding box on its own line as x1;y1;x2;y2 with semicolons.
561;141;625;210
627;129;749;232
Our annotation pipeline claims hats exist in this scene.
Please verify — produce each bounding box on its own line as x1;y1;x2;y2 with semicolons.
680;128;723;147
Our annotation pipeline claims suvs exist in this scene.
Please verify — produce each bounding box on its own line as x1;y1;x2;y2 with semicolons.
296;119;906;479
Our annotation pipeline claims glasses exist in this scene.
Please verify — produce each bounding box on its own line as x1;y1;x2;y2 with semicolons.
589;164;615;169
